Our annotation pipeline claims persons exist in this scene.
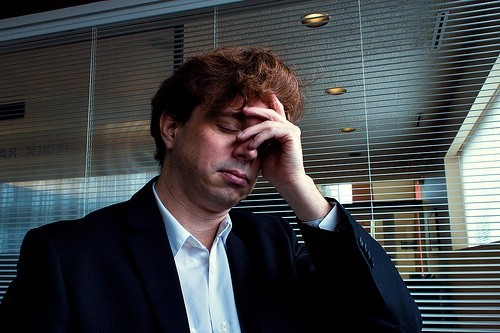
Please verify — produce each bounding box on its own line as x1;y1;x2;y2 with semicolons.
0;45;424;333
